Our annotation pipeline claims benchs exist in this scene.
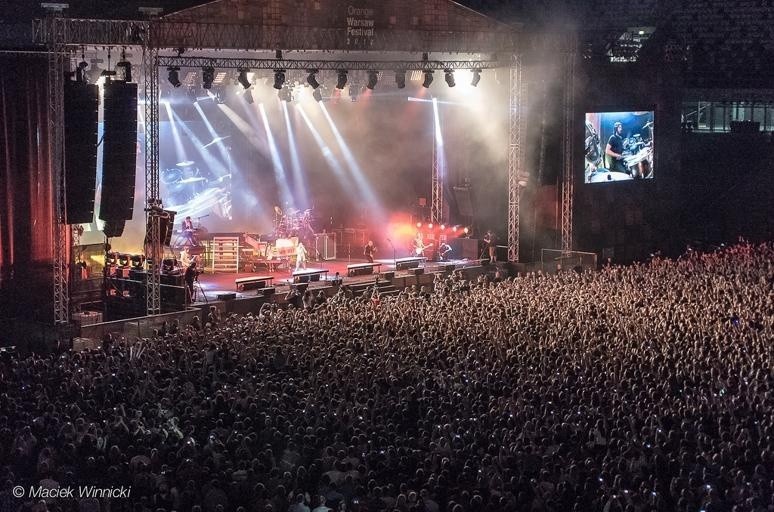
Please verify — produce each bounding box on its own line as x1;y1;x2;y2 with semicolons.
291;266;328;283
392;255;428;270
234;275;274;291
346;262;383;275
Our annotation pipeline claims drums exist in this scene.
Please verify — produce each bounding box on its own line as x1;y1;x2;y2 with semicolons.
625;153;653;179
276;237;299;255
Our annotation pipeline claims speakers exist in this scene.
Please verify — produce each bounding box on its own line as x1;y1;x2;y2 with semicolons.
62;81;99;225
239;281;266;290
99;77;138;237
524;69;563;188
348;265;374;277
144;211;177;247
453;186;480;217
396;261;419;270
294;273;320;283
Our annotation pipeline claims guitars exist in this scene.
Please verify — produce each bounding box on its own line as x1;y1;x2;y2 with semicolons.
416;243;432;254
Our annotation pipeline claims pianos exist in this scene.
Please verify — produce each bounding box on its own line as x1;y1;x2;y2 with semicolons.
174;229;196;232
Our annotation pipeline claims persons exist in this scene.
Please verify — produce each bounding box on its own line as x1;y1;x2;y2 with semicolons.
605;121;627;172
0;230;774;512
181;216;197;243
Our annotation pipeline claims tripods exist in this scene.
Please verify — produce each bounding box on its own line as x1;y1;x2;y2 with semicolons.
193;277;208;302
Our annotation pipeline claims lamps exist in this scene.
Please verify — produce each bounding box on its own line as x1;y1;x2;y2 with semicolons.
154;55;501;92
141;67;395;104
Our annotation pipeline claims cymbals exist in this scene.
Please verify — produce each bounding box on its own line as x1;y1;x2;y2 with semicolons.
642;122;653;129
275;207;282;215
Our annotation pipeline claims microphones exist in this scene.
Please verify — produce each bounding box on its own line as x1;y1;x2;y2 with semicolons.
387;238;392;241
